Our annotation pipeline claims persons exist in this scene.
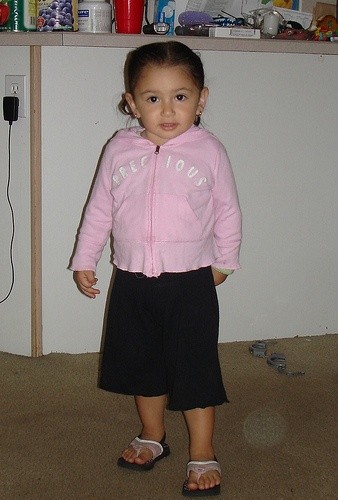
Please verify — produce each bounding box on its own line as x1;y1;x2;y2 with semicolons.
68;40;242;497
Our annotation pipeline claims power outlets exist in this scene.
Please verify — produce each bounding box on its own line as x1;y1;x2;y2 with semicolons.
5;75;27;118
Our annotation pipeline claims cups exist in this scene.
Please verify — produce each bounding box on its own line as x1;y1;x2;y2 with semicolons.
113;0;145;34
263;16;279;35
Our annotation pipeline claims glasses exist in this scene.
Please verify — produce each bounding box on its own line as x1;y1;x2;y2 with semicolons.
249;342;286;373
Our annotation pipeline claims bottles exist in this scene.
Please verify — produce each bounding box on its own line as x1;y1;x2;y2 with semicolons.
156;0;176;36
78;0;113;33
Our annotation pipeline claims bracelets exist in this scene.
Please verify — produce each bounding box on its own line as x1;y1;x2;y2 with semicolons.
213;267;234;277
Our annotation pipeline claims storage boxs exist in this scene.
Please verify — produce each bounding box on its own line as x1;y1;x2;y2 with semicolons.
209;27;260;39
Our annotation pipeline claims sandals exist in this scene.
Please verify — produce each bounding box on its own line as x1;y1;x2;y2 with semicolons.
118;431;169;471
183;455;221;497
